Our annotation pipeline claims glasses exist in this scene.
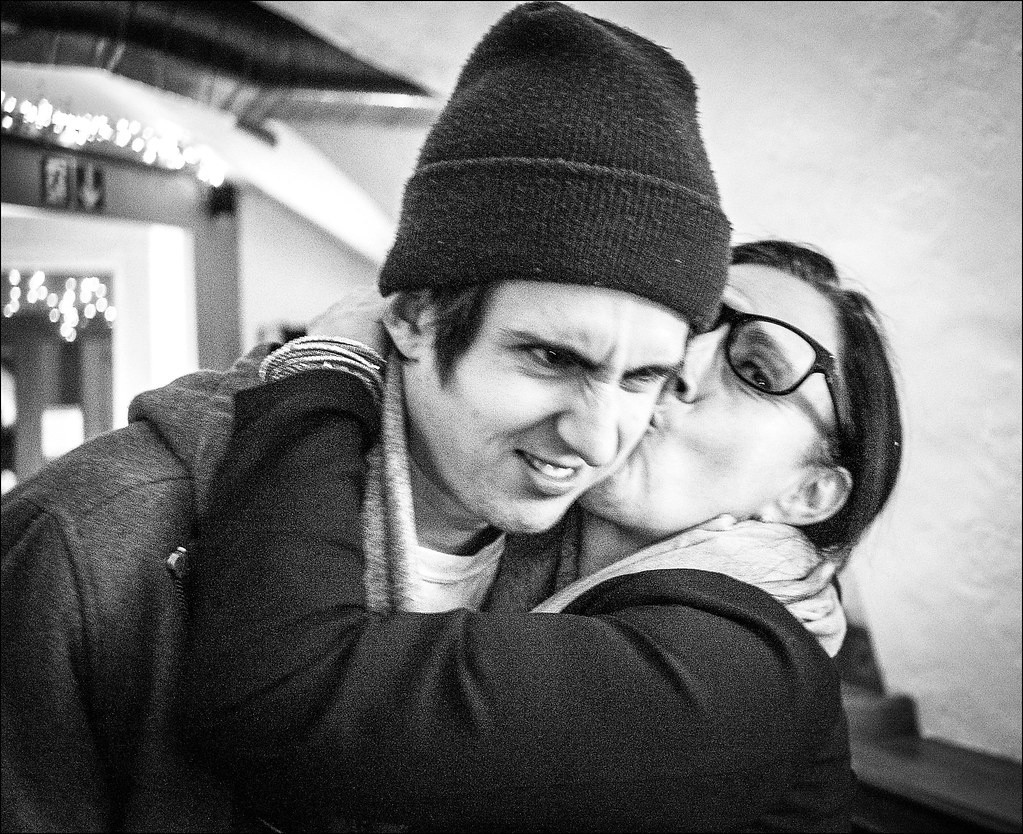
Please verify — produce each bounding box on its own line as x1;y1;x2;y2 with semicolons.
688;301;850;454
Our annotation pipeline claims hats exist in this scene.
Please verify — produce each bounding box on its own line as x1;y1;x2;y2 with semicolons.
374;0;734;334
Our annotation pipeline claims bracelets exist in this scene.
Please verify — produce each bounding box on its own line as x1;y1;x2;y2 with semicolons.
260;335;386;399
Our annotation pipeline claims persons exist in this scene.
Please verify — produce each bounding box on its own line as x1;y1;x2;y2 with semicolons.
182;238;904;834
0;1;734;834
